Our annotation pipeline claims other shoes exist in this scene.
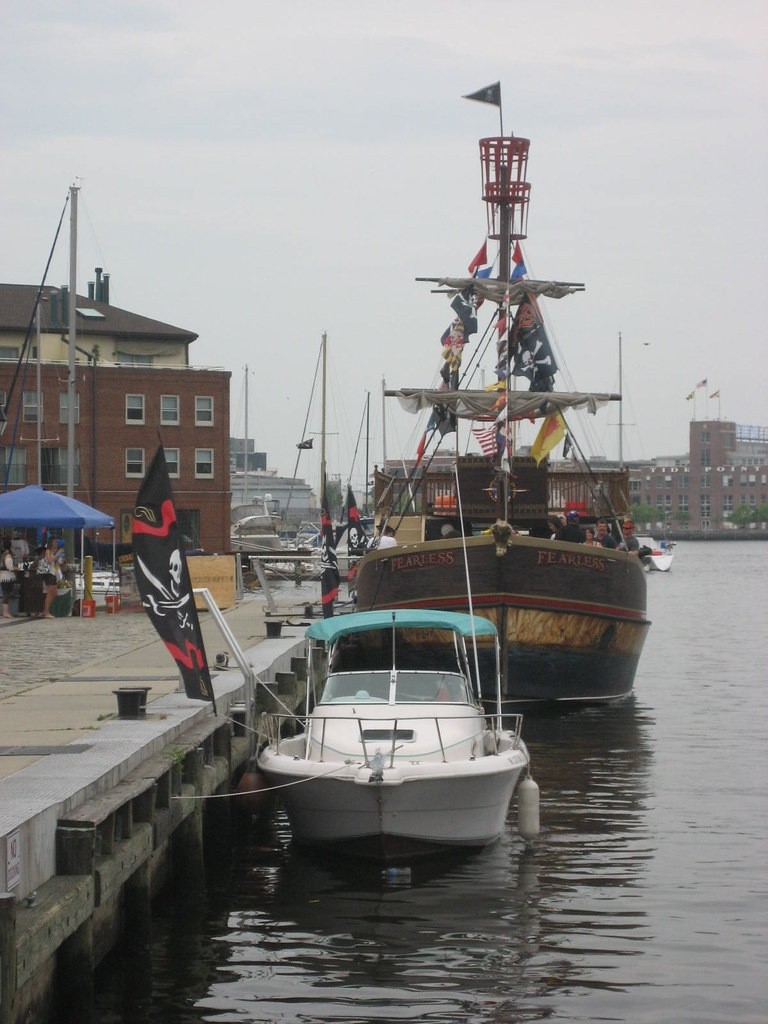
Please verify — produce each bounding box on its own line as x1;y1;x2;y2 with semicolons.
41;612;55;619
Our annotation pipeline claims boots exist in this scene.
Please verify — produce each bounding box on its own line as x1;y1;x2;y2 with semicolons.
2;604;12;618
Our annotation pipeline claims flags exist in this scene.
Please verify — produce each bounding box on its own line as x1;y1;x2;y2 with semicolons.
417;241;573;469
137;445;213;703
686;379;720;400
315;472;370;620
297;438;313;449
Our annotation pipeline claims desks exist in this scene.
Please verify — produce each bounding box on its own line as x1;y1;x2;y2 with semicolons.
49;587;72;618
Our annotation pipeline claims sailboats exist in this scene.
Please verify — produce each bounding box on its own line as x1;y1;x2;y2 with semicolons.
0;187;121;596
356;136;652;711
230;365;283;552
299;380;375;578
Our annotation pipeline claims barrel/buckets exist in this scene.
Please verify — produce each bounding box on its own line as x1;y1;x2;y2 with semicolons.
106;596;119;613
82;599;96;617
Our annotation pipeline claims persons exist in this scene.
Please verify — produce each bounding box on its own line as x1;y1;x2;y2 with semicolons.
0;541;21;618
548;510;639;556
42;537;63;618
367;525;398;551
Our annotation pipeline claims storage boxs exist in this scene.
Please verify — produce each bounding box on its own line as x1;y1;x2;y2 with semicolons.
566;500;585;518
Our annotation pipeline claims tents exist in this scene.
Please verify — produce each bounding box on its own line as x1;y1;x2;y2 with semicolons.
0;485;116;617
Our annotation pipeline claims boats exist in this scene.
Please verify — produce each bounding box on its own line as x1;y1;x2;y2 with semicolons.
257;609;529;846
633;533;674;572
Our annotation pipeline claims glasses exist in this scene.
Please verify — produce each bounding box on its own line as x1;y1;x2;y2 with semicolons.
623;526;632;530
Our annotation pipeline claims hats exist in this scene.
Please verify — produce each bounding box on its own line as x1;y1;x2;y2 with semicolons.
567;511;580;521
557;515;567;520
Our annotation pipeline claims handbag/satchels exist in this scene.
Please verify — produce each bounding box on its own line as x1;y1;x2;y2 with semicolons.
36;558;51;575
